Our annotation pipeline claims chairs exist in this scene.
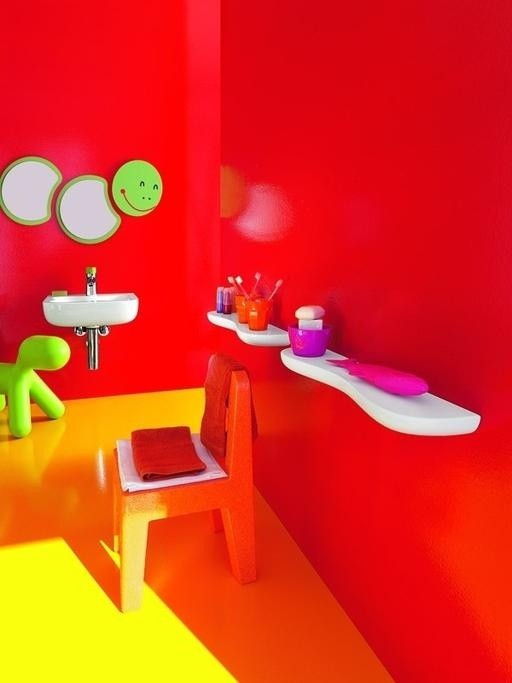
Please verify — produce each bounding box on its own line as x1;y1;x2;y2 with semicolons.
112;352;257;613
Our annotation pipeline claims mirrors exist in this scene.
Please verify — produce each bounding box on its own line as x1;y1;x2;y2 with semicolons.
1;155;63;227
55;173;122;247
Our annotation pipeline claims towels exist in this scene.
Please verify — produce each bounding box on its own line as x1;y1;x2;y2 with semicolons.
131;426;207;482
200;352;258;458
116;433;228;493
